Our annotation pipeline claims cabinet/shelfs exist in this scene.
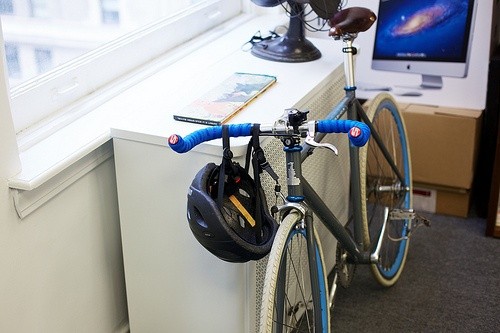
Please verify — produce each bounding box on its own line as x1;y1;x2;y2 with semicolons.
110;31;360;333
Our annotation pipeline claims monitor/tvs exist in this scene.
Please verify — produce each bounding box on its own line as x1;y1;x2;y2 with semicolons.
371;0;477;78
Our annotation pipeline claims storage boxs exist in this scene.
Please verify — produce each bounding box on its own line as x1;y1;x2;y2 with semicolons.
361;101;482;218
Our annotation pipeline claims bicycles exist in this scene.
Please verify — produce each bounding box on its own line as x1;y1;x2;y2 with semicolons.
168;6;431;333
